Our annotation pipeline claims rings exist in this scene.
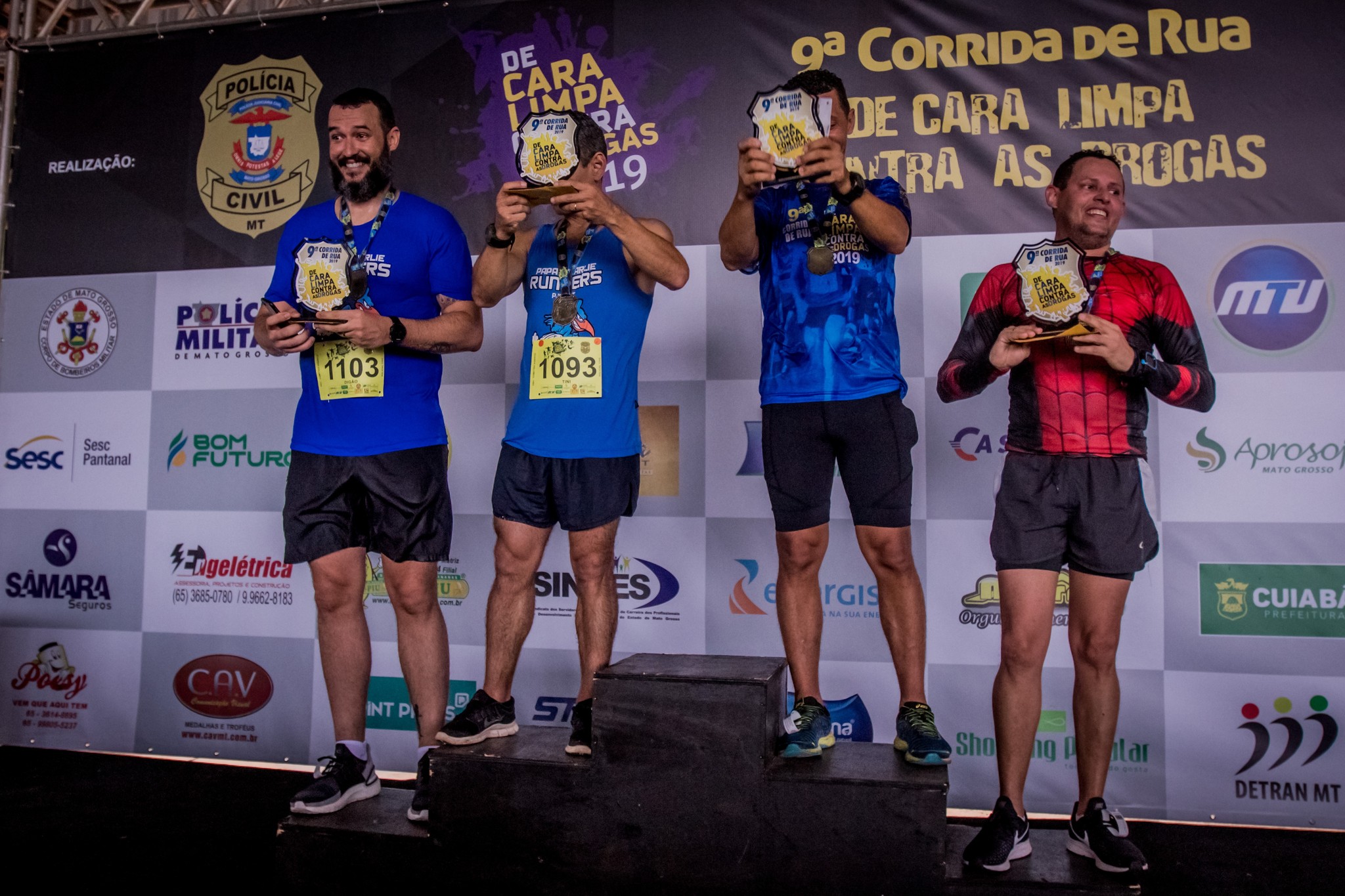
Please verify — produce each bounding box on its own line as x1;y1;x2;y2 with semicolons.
574;203;578;213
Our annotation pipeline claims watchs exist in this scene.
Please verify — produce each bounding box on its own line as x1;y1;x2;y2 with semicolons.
838;170;866;206
1131;350;1159;388
389;313;406;347
482;220;517;251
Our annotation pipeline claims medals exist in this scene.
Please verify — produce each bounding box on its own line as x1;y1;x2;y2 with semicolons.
806;244;835;276
551;293;578;327
1066;333;1086;351
351;268;367;300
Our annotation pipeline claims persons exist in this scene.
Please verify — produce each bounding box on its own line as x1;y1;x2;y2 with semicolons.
935;148;1219;875
431;110;687;757
713;68;955;771
244;89;455;828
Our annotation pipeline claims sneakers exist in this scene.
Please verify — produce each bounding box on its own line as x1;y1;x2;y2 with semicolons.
1066;796;1148;873
894;701;952;766
407;749;429;821
778;696;835;758
963;796;1033;872
565;697;591;754
435;689;519;744
290;743;381;814
